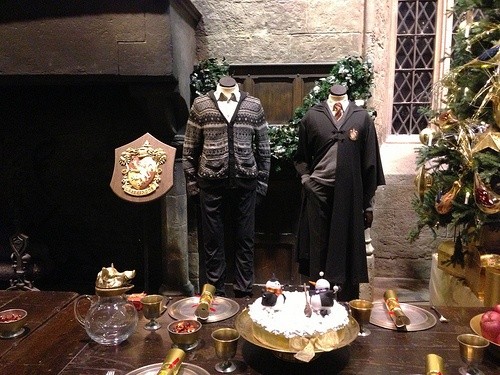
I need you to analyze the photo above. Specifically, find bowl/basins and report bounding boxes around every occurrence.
[470,313,500,346]
[236,306,360,359]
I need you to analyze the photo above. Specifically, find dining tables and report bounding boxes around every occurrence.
[0,295,500,375]
[0,288,77,357]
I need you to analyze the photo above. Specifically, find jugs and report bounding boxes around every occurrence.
[74,262,139,347]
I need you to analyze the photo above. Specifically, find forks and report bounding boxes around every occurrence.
[430,306,450,323]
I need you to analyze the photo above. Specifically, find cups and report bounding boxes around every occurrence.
[167,319,202,350]
[0,309,27,339]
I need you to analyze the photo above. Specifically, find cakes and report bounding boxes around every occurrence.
[248,271,349,350]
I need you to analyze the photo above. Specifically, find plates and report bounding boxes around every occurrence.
[125,362,211,375]
[167,295,239,323]
[369,302,437,332]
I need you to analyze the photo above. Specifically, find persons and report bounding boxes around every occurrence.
[294,85,386,302]
[182,77,270,297]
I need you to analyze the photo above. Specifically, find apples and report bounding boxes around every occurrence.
[480,304,500,344]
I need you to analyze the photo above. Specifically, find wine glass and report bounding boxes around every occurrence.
[211,328,240,373]
[455,334,489,374]
[139,295,163,330]
[349,300,374,335]
